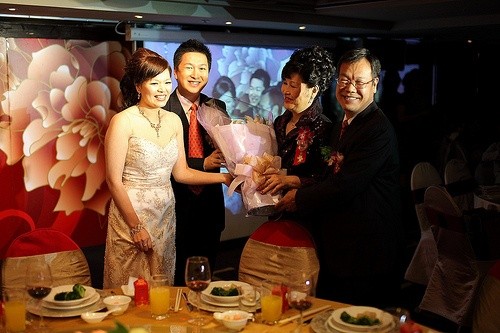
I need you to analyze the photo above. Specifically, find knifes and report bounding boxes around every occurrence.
[181,293,193,312]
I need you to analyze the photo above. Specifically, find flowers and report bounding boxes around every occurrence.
[195,94,288,216]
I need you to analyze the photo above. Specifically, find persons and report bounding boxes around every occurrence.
[103,47,242,292]
[255,44,423,314]
[161,38,226,289]
[211,68,285,128]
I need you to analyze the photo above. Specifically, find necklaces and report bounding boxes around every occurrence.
[135,100,162,140]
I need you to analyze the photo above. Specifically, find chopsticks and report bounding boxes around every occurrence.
[174,289,182,312]
[278,304,331,324]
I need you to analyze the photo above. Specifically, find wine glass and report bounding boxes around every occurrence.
[287,271,314,325]
[25,261,54,333]
[184,256,212,326]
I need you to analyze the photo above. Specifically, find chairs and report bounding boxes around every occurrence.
[1,227,91,304]
[238,220,321,297]
[411,143,500,333]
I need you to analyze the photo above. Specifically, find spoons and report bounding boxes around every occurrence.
[80,308,123,320]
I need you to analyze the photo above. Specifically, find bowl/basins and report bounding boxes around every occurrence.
[121,285,135,296]
[220,311,249,331]
[102,295,132,316]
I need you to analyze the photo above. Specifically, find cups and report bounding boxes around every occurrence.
[261,280,283,324]
[2,289,28,333]
[148,275,170,320]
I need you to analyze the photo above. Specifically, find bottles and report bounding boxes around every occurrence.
[134,273,149,308]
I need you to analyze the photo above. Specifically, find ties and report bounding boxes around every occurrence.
[187,104,204,195]
[342,119,348,134]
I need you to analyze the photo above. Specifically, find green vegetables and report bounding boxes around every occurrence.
[211,287,239,296]
[341,311,380,326]
[54,284,86,300]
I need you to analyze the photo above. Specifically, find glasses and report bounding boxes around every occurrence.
[335,75,374,89]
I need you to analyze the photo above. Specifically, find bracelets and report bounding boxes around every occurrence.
[131,224,142,237]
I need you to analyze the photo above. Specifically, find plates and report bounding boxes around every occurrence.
[310,305,403,333]
[188,280,273,313]
[25,284,105,318]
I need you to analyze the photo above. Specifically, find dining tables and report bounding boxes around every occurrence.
[0,279,438,333]
